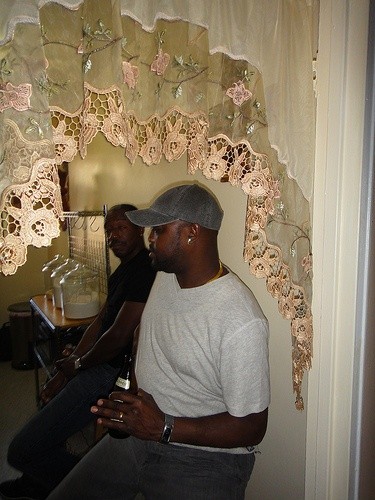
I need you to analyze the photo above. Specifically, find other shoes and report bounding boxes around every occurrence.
[0,475,51,500]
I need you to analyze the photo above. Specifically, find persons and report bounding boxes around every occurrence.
[0,204,156,499]
[43,184,270,500]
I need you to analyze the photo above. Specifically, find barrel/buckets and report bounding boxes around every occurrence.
[6,302,42,370]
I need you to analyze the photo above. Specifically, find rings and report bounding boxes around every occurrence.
[119,412,124,420]
[39,383,49,393]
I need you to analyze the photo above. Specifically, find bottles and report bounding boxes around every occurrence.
[109,353,138,439]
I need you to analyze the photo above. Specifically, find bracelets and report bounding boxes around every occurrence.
[74,357,82,370]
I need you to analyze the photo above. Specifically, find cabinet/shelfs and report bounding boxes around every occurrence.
[28,205,111,409]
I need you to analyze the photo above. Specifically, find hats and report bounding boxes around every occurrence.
[124,183,224,231]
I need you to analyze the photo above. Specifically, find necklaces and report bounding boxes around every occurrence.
[201,259,225,286]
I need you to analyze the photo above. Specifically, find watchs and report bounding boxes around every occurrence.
[160,414,175,445]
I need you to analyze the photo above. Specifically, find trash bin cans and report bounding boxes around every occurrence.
[5,301,40,371]
